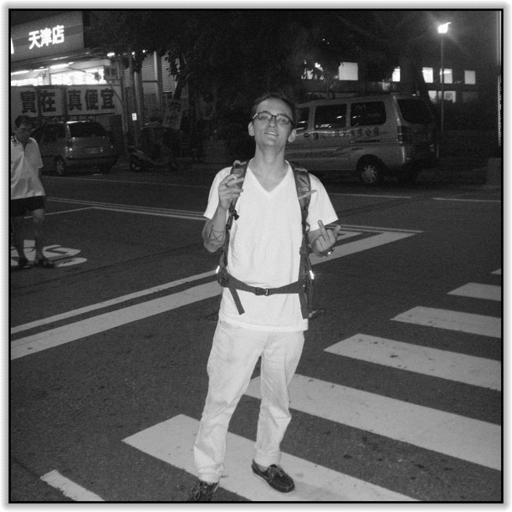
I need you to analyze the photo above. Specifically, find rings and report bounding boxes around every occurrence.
[224,181,231,191]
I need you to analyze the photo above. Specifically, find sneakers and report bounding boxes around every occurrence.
[17,254,54,269]
[187,478,219,502]
[251,459,294,492]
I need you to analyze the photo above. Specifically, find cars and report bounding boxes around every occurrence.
[30,120,118,175]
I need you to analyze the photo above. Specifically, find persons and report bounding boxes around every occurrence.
[185,95,340,501]
[12,114,51,272]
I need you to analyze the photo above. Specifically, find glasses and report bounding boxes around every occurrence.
[252,110,295,129]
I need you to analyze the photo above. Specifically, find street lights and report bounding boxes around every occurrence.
[438,22,451,134]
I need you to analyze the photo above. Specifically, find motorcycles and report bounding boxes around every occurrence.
[126,131,178,175]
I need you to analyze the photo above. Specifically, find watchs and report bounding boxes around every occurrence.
[323,247,334,259]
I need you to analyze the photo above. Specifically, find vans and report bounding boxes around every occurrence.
[284,93,437,187]
